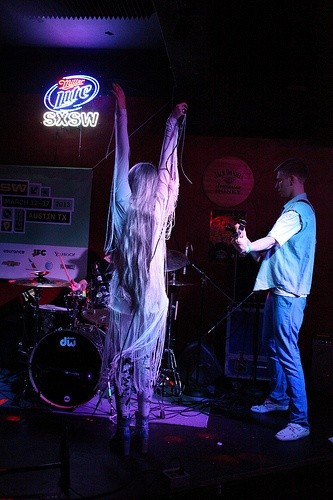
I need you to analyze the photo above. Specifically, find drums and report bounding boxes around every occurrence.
[30,323,105,408]
[17,305,78,355]
[80,280,111,325]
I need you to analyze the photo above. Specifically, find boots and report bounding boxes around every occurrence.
[131,412,150,440]
[110,417,130,452]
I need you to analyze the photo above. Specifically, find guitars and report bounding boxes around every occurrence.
[225,219,269,297]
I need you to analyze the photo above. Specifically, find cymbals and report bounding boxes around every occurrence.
[168,281,195,286]
[104,249,189,272]
[8,271,71,288]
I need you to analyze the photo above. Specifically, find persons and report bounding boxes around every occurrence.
[230,160,316,440]
[48,249,106,308]
[104,81,188,460]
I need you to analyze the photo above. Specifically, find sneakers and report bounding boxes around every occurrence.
[250,399,289,413]
[275,423,310,441]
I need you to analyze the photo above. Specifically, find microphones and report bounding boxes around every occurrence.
[182,244,189,275]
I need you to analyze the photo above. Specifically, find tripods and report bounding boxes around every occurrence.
[163,286,184,406]
